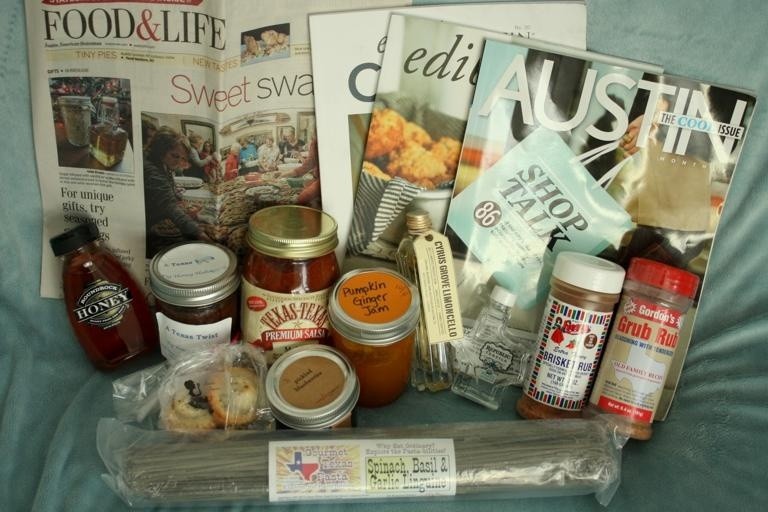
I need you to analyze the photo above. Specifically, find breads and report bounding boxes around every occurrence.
[163,367,259,435]
[363,108,463,189]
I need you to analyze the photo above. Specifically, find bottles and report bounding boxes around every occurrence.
[87,96,127,169]
[450,284,531,411]
[513,249,628,419]
[52,202,471,433]
[582,257,700,442]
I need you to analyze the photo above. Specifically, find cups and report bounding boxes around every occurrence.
[55,93,94,149]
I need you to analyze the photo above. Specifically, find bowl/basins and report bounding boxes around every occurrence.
[282,157,300,165]
[277,163,302,172]
[378,188,453,246]
[287,177,305,188]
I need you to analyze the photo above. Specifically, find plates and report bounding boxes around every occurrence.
[172,176,204,188]
[180,189,213,201]
[243,184,281,198]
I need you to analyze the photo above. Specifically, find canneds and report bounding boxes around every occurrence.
[326,266,421,408]
[239,203,339,370]
[264,344,359,433]
[147,237,240,365]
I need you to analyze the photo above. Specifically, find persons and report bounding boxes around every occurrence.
[144,116,319,247]
